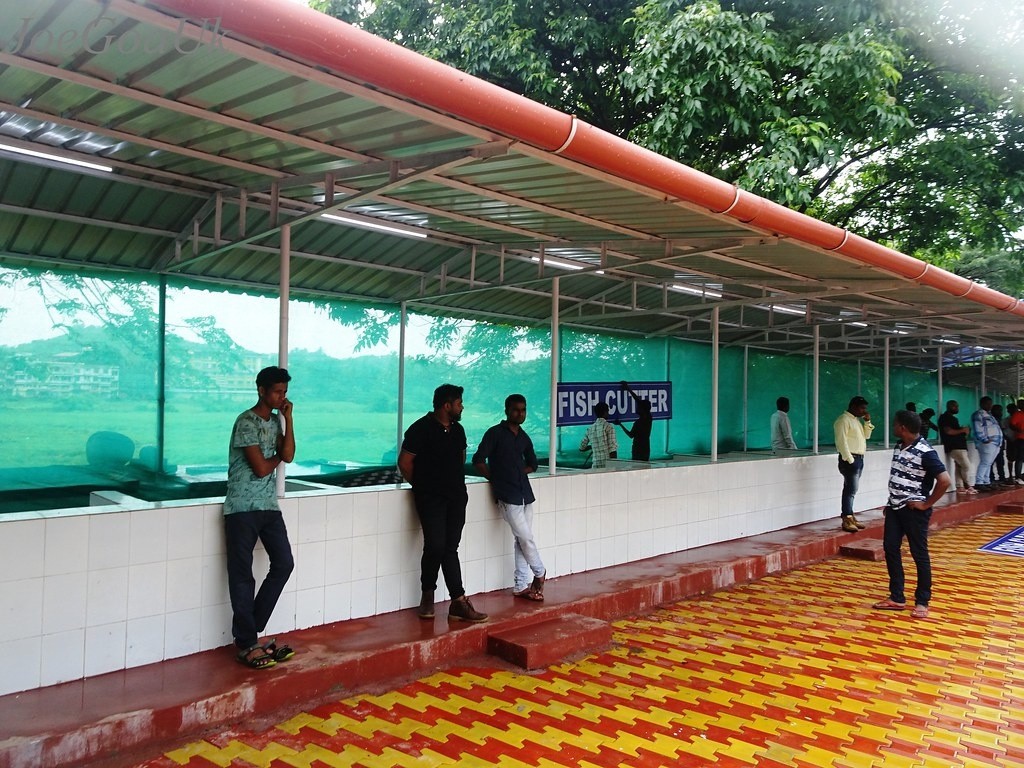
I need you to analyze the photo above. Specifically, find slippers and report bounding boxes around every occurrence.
[515,589,545,601]
[956,488,968,494]
[531,573,545,597]
[966,488,979,495]
[873,599,906,610]
[911,603,927,617]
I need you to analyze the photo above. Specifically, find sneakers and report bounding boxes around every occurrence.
[842,516,858,532]
[418,590,435,617]
[852,516,865,529]
[448,600,489,623]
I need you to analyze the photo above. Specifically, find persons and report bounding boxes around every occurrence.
[937,400,978,494]
[397,384,488,622]
[579,402,618,469]
[223,366,295,668]
[972,396,1024,491]
[613,381,652,460]
[833,396,875,532]
[471,393,546,600]
[906,401,916,413]
[874,410,951,616]
[770,397,798,454]
[918,408,939,440]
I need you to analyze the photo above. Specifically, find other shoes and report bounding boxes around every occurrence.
[975,478,1024,491]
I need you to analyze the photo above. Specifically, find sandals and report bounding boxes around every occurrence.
[236,644,277,669]
[263,642,294,661]
